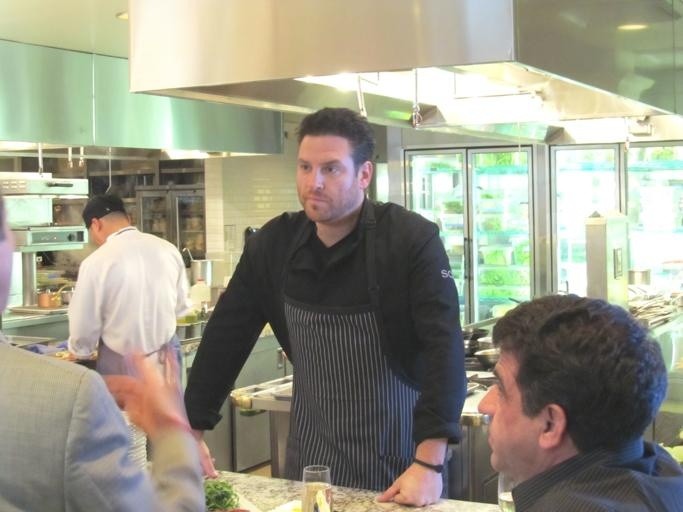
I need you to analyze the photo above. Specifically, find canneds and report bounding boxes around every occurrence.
[48,292,62,308]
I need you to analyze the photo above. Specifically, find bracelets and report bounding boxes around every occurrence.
[411,456,445,473]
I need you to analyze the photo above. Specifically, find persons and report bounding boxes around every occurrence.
[178,104,468,509]
[0,196,205,512]
[55,195,192,400]
[476,293,681,512]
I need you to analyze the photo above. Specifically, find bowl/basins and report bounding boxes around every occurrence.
[461,326,501,367]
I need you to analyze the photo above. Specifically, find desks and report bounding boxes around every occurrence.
[203,470,502,512]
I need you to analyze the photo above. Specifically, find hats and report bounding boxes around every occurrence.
[81,195,124,229]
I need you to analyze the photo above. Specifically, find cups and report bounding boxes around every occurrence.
[497,471,518,511]
[37,284,74,308]
[302,464,333,512]
[190,259,213,290]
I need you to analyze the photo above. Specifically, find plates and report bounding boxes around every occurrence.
[121,412,150,475]
